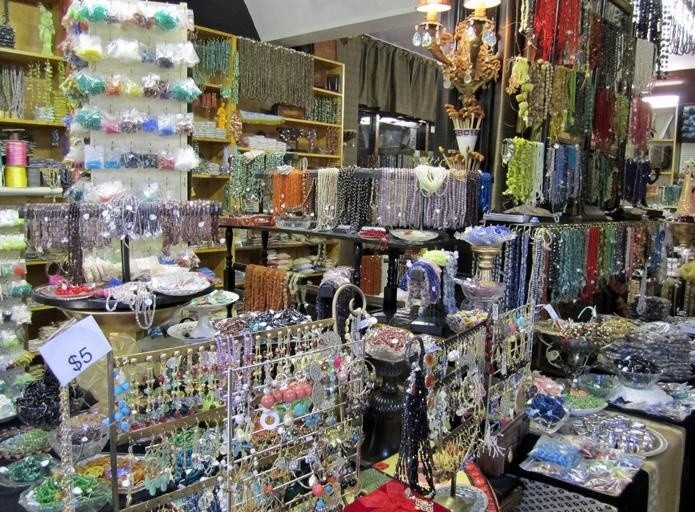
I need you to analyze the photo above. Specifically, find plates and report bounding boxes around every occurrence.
[568,402,610,417]
[390,228,439,245]
[0,454,60,489]
[167,321,222,344]
[634,426,669,457]
[32,283,94,301]
[429,483,489,512]
[147,281,211,296]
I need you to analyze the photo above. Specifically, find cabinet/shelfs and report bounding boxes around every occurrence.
[0,1,81,286]
[185,24,347,288]
[219,149,494,337]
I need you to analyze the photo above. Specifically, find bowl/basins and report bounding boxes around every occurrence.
[612,368,664,389]
[577,373,620,399]
[14,397,84,430]
[47,422,110,461]
[18,474,113,512]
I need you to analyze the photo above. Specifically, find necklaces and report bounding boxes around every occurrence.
[0,1,694,512]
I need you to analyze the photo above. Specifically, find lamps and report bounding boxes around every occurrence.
[413,0,509,171]
